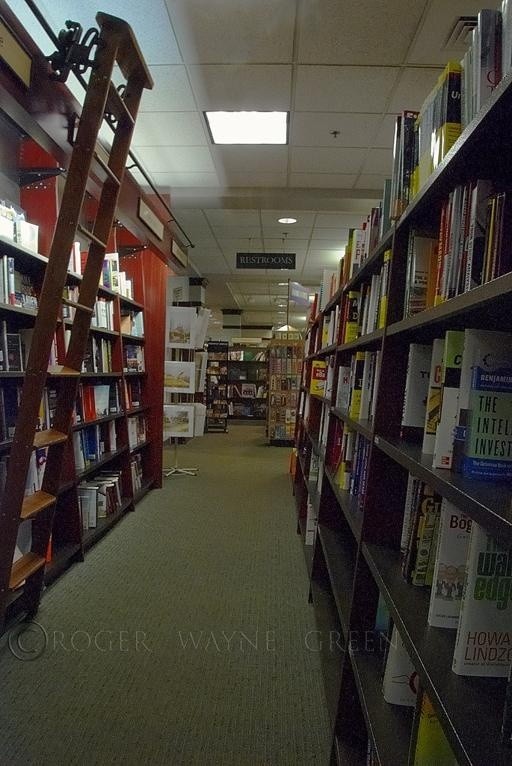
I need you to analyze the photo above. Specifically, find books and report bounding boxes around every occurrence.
[163,305,305,442]
[288,0,512,764]
[0,200,149,595]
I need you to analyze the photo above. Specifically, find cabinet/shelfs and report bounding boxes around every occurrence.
[1,1,229,633]
[266,68,512,765]
[227,346,268,417]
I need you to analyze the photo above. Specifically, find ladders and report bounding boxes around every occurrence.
[0,11,154,636]
